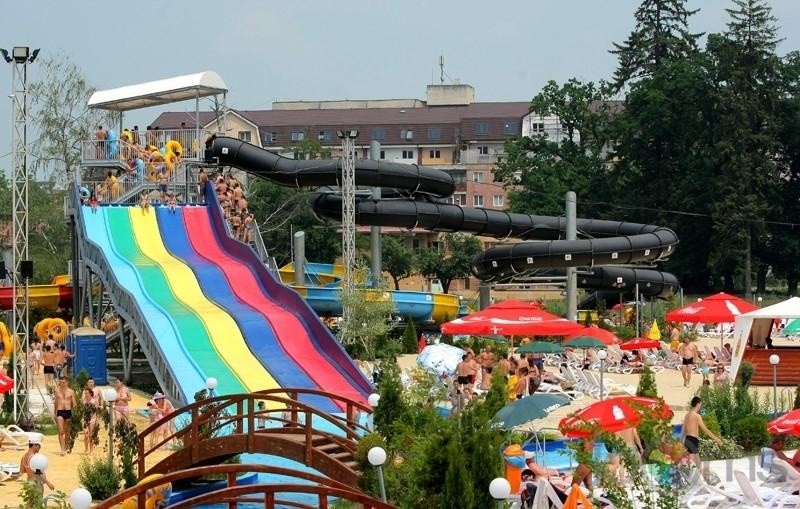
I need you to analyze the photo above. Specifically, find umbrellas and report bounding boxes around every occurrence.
[487,392,573,473]
[667,291,760,363]
[515,324,660,378]
[439,296,585,359]
[768,407,800,438]
[783,319,799,336]
[560,396,672,482]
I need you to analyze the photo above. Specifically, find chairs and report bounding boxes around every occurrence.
[550,365,628,403]
[0,425,46,451]
[0,462,25,484]
[519,446,799,509]
[542,337,731,376]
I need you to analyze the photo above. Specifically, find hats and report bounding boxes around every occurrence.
[151,392,166,400]
[28,439,42,449]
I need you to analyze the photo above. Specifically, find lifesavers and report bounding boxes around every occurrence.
[120,130,182,183]
[34,317,67,343]
[120,474,173,508]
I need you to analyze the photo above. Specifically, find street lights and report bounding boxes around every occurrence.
[769,354,780,421]
[69,487,93,509]
[596,346,606,407]
[0,46,43,431]
[104,383,118,479]
[29,453,49,490]
[489,475,511,509]
[459,295,464,306]
[757,294,762,308]
[364,445,388,505]
[335,127,359,349]
[365,391,379,436]
[205,377,217,398]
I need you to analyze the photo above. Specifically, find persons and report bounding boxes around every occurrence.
[19,333,176,508]
[521,395,724,509]
[558,305,734,392]
[451,338,546,415]
[281,401,304,428]
[257,401,271,428]
[81,120,255,247]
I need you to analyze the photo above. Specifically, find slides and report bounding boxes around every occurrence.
[280,264,477,332]
[81,203,379,419]
[206,133,680,311]
[0,272,97,310]
